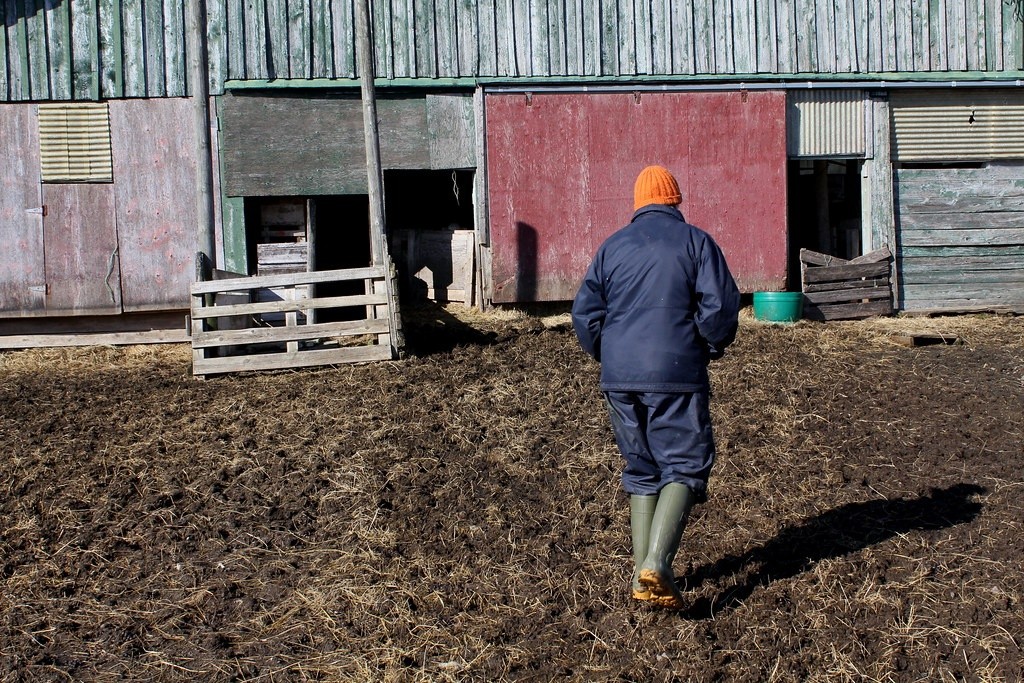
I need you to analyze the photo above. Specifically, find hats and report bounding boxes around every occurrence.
[633,166,682,212]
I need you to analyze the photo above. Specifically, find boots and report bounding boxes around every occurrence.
[638,482,689,607]
[631,493,658,600]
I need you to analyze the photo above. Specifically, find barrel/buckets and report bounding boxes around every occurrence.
[753,291,804,323]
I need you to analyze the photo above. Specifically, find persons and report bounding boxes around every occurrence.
[570,166,741,608]
